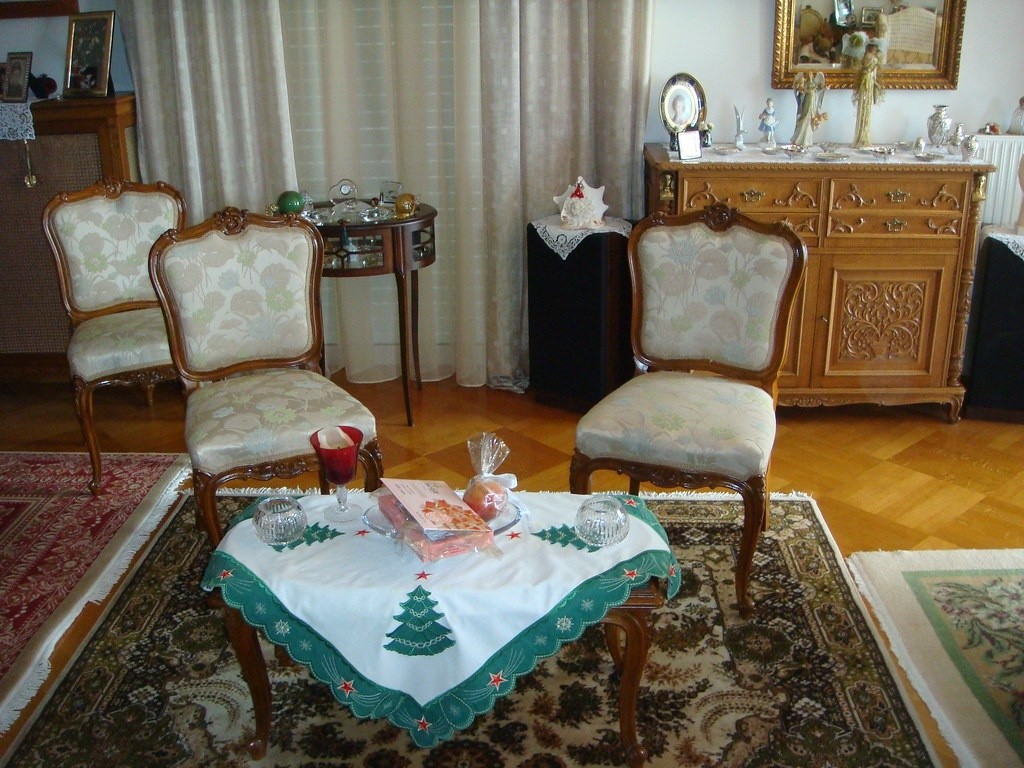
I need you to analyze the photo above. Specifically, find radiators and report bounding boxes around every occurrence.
[966,131,1024,225]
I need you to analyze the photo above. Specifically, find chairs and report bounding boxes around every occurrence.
[42,177,206,493]
[568,201,808,623]
[879,6,943,66]
[149,206,386,549]
[799,8,823,41]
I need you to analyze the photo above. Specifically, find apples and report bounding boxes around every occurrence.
[463,481,506,521]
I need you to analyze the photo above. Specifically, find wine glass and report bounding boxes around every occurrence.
[309,425,364,521]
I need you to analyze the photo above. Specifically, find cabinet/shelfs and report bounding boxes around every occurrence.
[643,143,997,423]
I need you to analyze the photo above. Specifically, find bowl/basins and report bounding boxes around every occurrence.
[818,141,843,152]
[870,147,899,160]
[781,144,810,160]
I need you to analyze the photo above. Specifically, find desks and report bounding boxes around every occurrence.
[526,213,636,416]
[0,90,141,384]
[199,487,681,766]
[273,198,437,429]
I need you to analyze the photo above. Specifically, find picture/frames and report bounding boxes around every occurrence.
[3,52,43,103]
[862,7,884,25]
[657,71,712,152]
[770,0,967,90]
[63,10,116,99]
[0,63,7,100]
[833,0,854,24]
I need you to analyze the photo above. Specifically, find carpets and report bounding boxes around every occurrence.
[0,448,192,737]
[0,480,942,768]
[844,547,1024,768]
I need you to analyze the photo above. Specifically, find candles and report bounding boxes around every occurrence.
[310,425,365,522]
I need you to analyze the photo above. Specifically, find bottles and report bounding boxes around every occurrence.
[912,138,925,154]
[574,496,630,547]
[962,134,979,162]
[927,105,952,148]
[252,496,308,545]
[947,124,965,155]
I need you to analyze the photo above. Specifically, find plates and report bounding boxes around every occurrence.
[714,147,741,154]
[914,153,944,162]
[763,147,783,155]
[362,500,521,539]
[815,152,851,162]
